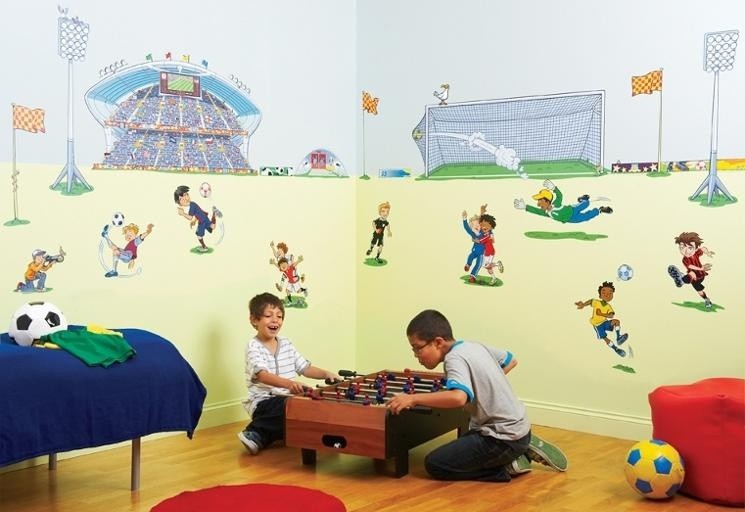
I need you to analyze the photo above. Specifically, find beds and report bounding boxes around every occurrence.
[0,325,208,492]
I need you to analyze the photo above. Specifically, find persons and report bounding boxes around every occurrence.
[575,281,629,357]
[269,241,305,283]
[276,255,308,307]
[15,249,56,292]
[668,232,716,308]
[174,186,223,252]
[366,202,393,264]
[472,214,503,285]
[386,310,569,483]
[101,223,153,277]
[462,203,495,283]
[514,180,613,223]
[237,292,342,457]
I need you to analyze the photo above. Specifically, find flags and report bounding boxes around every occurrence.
[632,71,662,96]
[12,104,45,134]
[146,52,208,67]
[361,91,380,115]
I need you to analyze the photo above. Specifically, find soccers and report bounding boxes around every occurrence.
[113,215,125,226]
[15,302,65,345]
[201,184,211,199]
[618,265,634,281]
[625,439,685,501]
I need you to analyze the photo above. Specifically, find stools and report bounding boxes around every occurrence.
[149,483,346,512]
[648,378,744,507]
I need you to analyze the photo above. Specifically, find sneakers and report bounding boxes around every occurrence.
[504,454,533,476]
[526,433,568,472]
[237,429,263,455]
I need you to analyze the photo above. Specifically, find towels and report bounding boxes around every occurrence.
[40,329,136,369]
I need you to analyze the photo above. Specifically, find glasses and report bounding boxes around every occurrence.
[412,341,431,354]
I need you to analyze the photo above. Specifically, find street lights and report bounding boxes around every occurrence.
[689,30,739,204]
[50,16,92,194]
